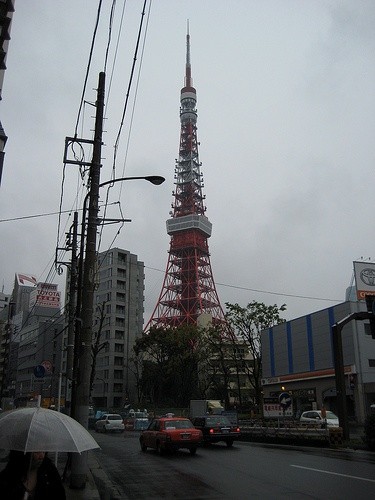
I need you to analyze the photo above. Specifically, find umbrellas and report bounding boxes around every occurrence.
[0,394,101,500]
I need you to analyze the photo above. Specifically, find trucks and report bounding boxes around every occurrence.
[189,400,225,417]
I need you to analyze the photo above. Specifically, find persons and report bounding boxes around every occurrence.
[0,432,66,500]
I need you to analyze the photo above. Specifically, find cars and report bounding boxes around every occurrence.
[139,413,203,457]
[299,410,339,428]
[87,404,150,430]
[95,413,125,433]
[191,416,241,449]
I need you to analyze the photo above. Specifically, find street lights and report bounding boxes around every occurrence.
[63,175,166,483]
